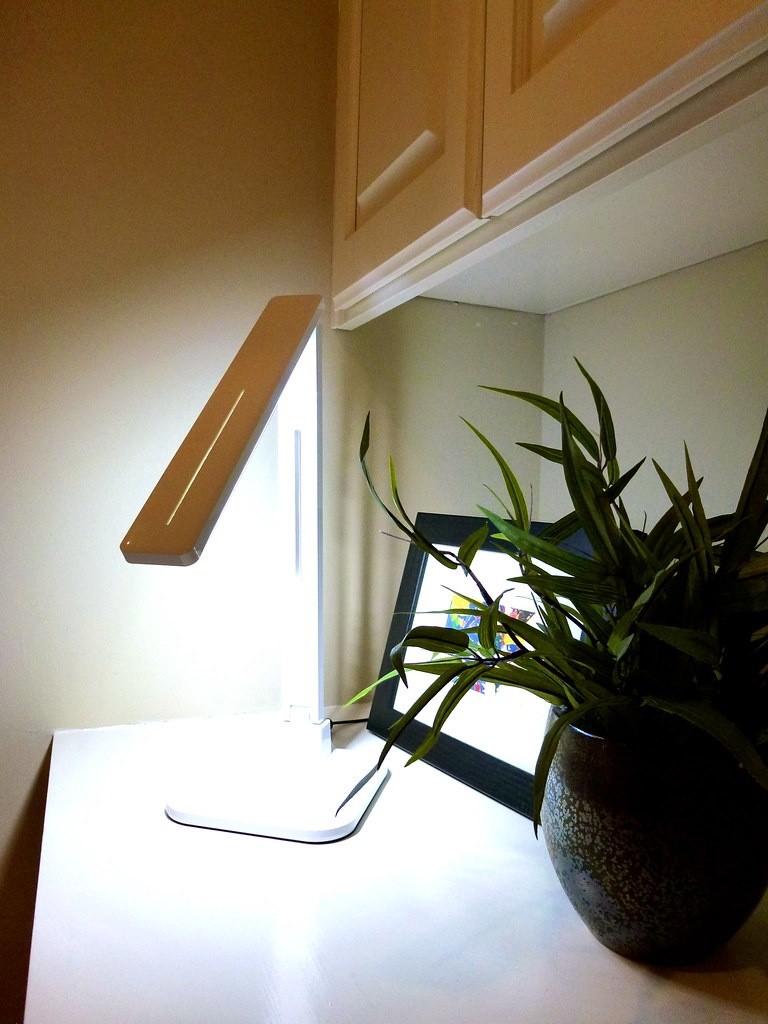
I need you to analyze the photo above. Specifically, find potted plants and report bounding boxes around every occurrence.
[314,353,768,976]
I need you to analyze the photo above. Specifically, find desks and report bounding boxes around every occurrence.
[23,717,768,1024]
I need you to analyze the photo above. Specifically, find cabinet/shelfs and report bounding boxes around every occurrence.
[325,0,768,334]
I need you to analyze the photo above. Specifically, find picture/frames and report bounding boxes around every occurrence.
[364,511,573,826]
